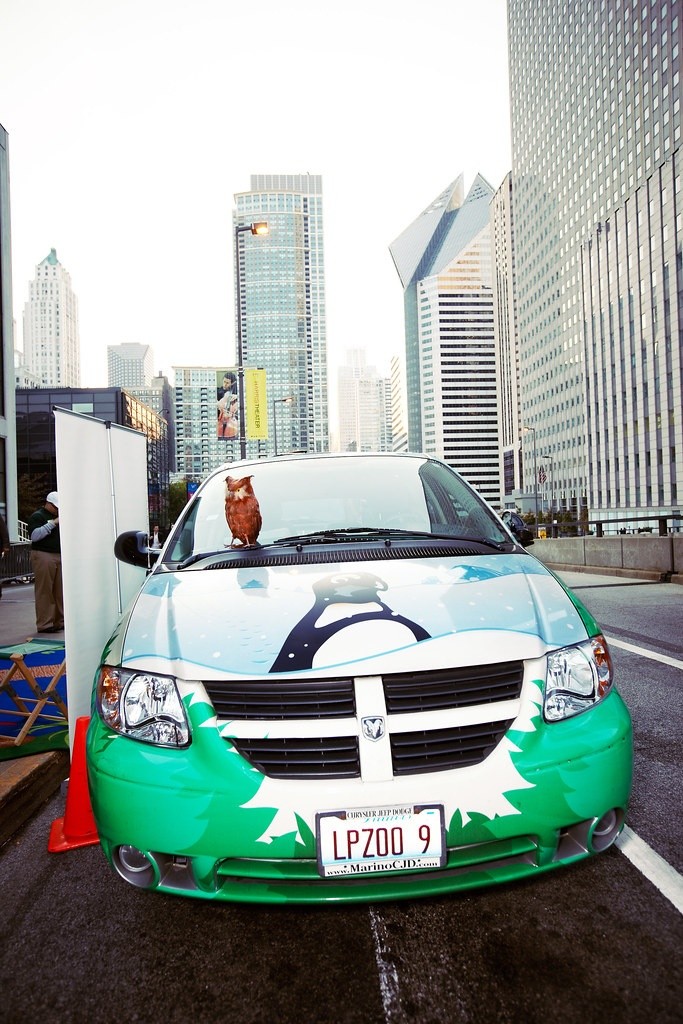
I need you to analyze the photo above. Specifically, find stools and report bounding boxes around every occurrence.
[0,638,68,747]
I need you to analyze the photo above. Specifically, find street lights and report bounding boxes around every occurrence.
[542,456,553,538]
[235,222,268,460]
[524,427,538,538]
[273,398,293,457]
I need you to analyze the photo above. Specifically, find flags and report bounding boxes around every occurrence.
[538,466,547,484]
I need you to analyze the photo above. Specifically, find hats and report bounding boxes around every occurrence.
[47,491,58,508]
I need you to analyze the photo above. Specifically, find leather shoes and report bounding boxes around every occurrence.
[57,627,64,630]
[38,626,62,633]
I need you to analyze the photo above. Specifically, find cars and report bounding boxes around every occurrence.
[85,452,634,903]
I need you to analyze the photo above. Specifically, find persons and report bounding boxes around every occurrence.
[149,523,175,568]
[620,526,652,534]
[0,514,10,598]
[217,373,239,439]
[29,491,65,634]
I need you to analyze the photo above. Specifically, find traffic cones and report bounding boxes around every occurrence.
[48,715,101,852]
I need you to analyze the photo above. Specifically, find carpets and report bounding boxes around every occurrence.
[0,643,70,761]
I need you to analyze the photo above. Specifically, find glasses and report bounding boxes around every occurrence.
[50,502,58,509]
